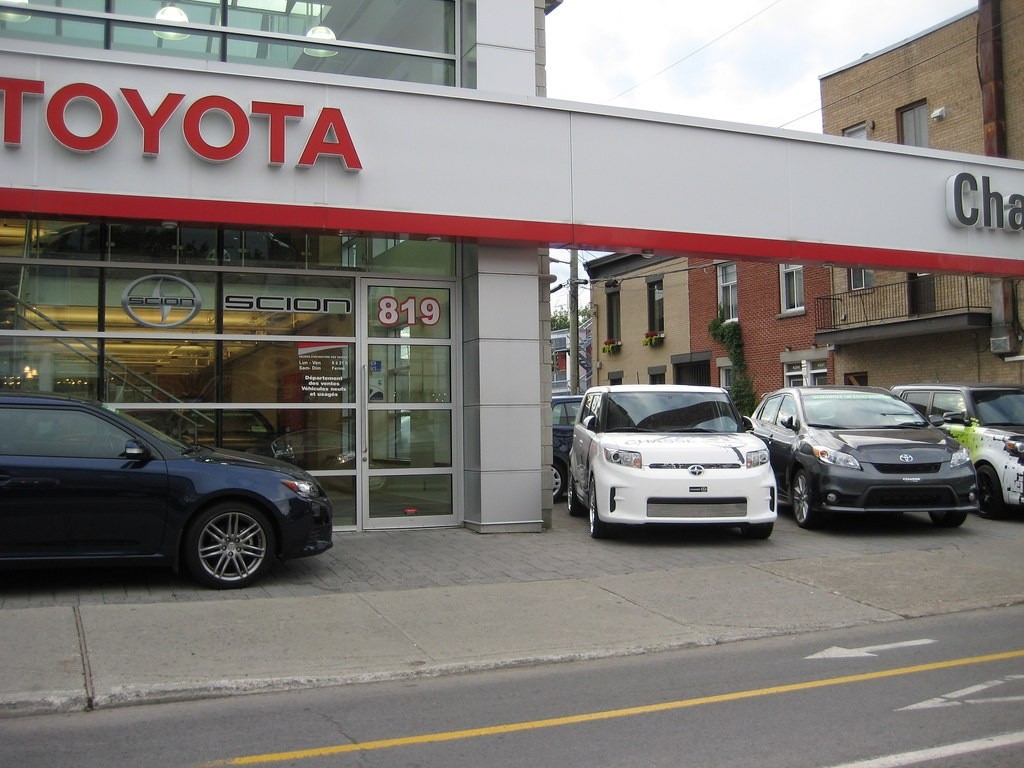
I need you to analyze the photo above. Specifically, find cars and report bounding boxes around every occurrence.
[172,409,294,464]
[551,395,585,503]
[889,384,1024,520]
[1,390,334,591]
[567,384,778,541]
[80,225,295,260]
[746,385,981,531]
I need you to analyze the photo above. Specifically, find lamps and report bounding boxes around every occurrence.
[302,0,339,58]
[152,0,191,42]
[930,108,946,122]
[0,1,32,23]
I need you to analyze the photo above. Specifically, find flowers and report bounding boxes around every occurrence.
[602,340,618,352]
[641,331,661,347]
